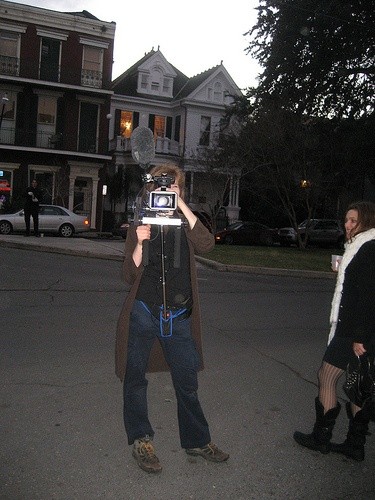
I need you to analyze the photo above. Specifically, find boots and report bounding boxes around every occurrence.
[328,401,371,462]
[293,396,341,455]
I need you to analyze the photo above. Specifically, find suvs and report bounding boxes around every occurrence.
[277,218,345,250]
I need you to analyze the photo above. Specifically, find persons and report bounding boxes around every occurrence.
[22,179,42,237]
[114,165,230,473]
[294,201,375,462]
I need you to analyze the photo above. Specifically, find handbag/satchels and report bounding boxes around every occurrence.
[342,357,375,407]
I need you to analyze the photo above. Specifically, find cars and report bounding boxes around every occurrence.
[0,204,90,235]
[112,210,213,240]
[214,221,278,247]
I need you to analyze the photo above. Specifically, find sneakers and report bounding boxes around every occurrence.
[186,442,230,462]
[132,435,162,473]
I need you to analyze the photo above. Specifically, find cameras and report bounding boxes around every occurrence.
[144,173,178,211]
